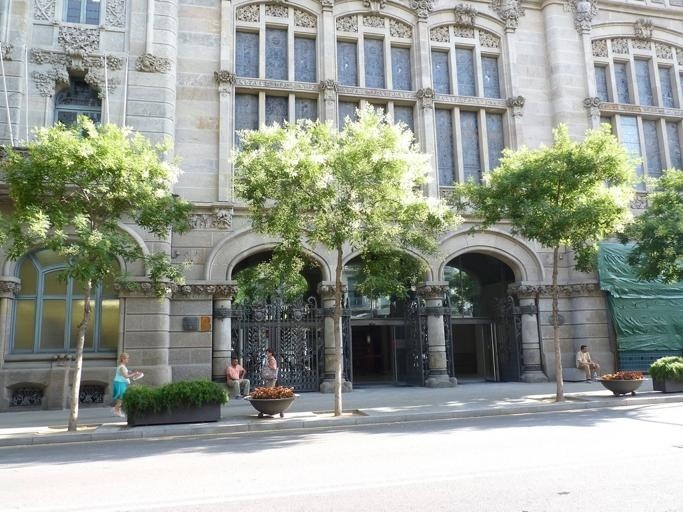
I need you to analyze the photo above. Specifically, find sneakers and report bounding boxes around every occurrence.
[234,395,251,400]
[587,379,594,384]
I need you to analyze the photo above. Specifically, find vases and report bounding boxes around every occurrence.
[245,397,299,417]
[598,379,648,393]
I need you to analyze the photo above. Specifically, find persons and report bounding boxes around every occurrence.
[265,348,279,387]
[226,356,250,399]
[113,352,140,417]
[576,345,601,383]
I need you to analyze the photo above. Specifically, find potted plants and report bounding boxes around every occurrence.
[121,380,226,426]
[649,356,683,392]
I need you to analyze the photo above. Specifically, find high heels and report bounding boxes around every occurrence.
[112,407,126,418]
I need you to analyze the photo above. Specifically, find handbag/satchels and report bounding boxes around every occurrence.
[262,367,278,379]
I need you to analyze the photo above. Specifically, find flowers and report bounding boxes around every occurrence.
[250,385,297,398]
[601,370,644,380]
